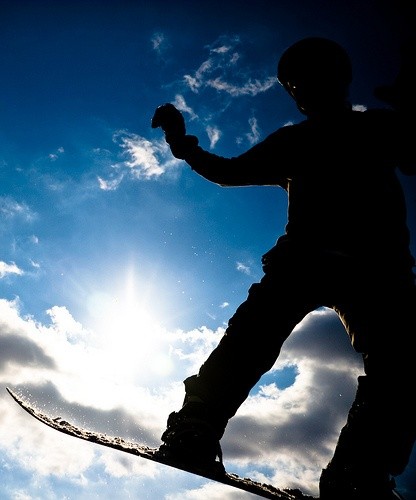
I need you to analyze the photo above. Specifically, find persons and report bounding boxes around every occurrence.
[149,33,416,500]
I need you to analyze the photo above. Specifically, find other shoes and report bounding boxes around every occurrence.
[164,408,228,471]
[320,465,407,500]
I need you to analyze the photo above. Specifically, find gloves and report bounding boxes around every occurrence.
[150,103,186,145]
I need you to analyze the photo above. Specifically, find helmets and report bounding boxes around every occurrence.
[278,37,353,87]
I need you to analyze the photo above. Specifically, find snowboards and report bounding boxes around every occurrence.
[5,386,416,500]
